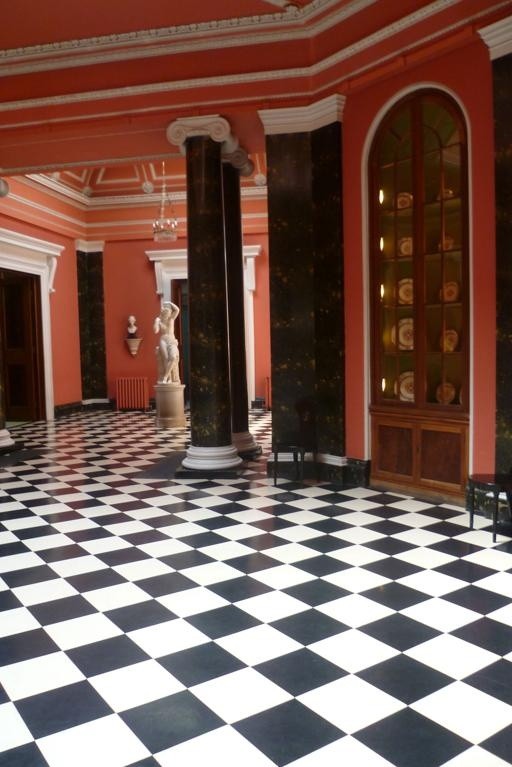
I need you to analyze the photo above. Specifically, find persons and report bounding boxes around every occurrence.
[127,316,137,333]
[153,301,181,384]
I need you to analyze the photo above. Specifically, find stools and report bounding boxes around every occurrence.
[467,473,512,542]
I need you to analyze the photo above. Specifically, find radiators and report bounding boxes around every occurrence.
[116,376,149,412]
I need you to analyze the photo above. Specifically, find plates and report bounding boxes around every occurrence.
[388,187,465,405]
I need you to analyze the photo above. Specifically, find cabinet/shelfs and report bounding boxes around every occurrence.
[360,81,473,499]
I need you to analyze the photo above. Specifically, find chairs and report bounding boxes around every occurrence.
[274,400,318,487]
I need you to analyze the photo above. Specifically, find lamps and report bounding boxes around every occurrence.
[152,160,178,243]
